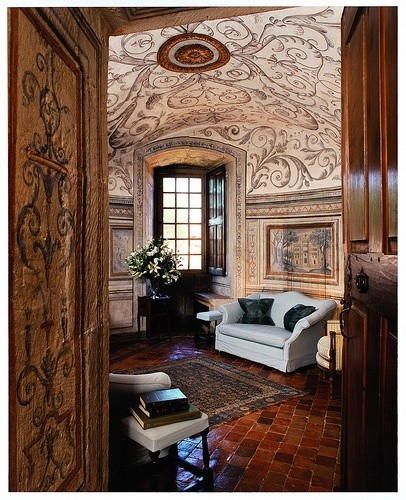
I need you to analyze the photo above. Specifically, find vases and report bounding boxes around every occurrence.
[151,285,170,298]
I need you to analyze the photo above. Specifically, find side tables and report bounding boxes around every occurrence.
[123,411,218,492]
[196,311,220,349]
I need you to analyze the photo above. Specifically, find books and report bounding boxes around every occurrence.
[128,387,202,431]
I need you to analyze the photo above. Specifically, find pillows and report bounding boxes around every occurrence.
[284,303,315,332]
[237,297,275,326]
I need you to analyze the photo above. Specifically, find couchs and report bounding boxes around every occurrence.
[109,372,172,469]
[215,290,336,374]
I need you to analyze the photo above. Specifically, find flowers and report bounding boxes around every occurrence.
[123,237,184,299]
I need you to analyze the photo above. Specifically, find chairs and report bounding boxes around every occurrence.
[316,319,344,400]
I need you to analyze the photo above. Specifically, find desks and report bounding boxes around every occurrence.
[194,292,229,334]
[137,297,175,346]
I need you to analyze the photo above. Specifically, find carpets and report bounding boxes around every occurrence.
[115,356,308,439]
